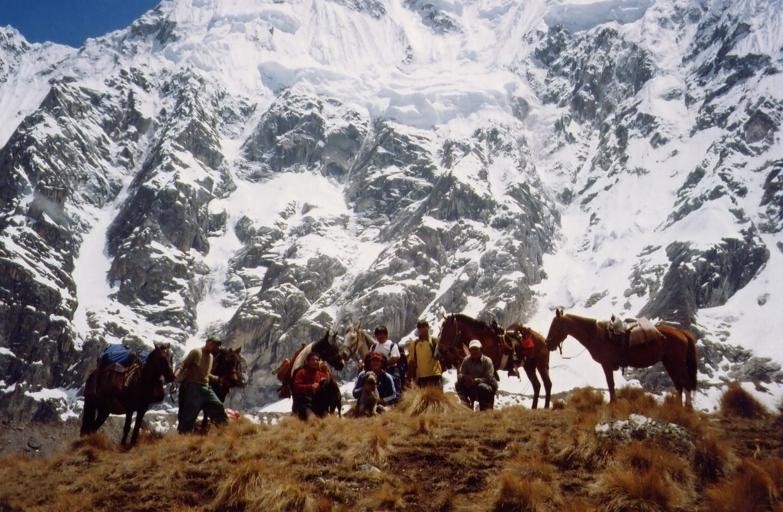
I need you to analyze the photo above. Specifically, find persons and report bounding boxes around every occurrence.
[454,339,499,412]
[173,335,230,435]
[370,325,401,393]
[292,351,331,427]
[352,351,401,405]
[407,318,444,394]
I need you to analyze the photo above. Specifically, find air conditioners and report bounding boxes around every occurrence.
[354,370,387,419]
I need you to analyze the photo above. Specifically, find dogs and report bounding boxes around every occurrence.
[342,321,410,384]
[193,346,245,434]
[545,308,697,408]
[276,329,344,418]
[433,311,552,411]
[79,341,176,449]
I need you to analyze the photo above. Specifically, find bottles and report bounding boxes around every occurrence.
[369,350,383,361]
[206,335,224,346]
[468,338,483,351]
[375,326,389,338]
